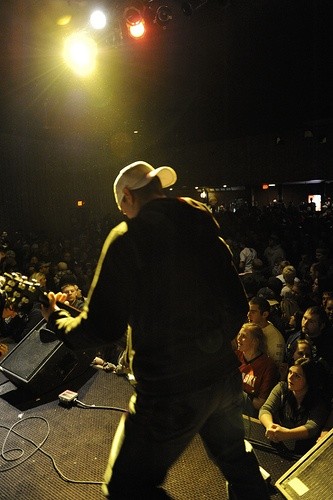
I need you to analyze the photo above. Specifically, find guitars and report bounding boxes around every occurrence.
[0,273,83,316]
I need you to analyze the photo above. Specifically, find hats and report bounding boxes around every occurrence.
[257,286,279,307]
[112,160,176,212]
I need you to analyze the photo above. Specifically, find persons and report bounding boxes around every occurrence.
[38,161,272,500]
[258,357,328,443]
[0,178,332,390]
[231,322,277,419]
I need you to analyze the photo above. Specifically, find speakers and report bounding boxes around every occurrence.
[275,428,333,500]
[0,317,86,395]
